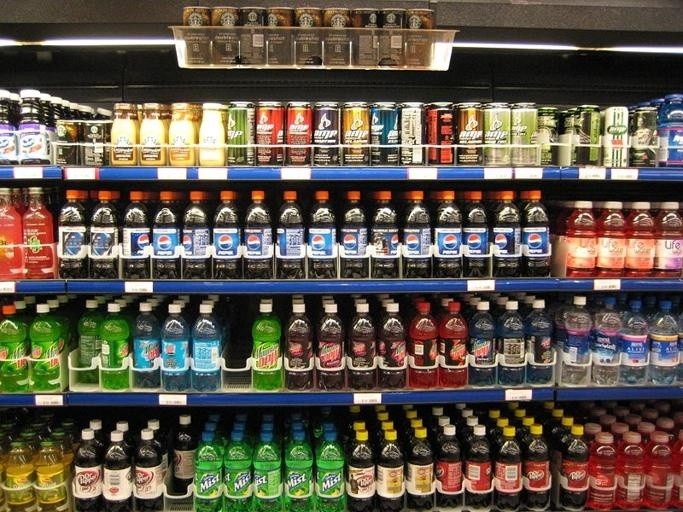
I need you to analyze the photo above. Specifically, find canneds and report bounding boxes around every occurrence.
[372,102,400,168]
[602,105,630,168]
[398,102,426,165]
[225,101,256,165]
[313,101,340,166]
[483,102,511,166]
[426,101,455,164]
[558,108,580,168]
[650,98,665,107]
[577,104,602,167]
[511,102,538,168]
[285,101,314,165]
[54,120,107,166]
[638,100,650,107]
[255,101,285,167]
[456,101,484,165]
[538,107,559,166]
[341,101,371,166]
[184,7,433,66]
[659,94,683,167]
[631,106,656,166]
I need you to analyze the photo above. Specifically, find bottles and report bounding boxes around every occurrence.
[255,296,683,393]
[1,295,220,389]
[0,404,682,511]
[0,87,229,168]
[628,94,681,169]
[0,185,683,285]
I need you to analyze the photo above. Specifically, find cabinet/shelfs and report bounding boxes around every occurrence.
[0,162,68,415]
[554,161,682,405]
[50,153,565,420]
